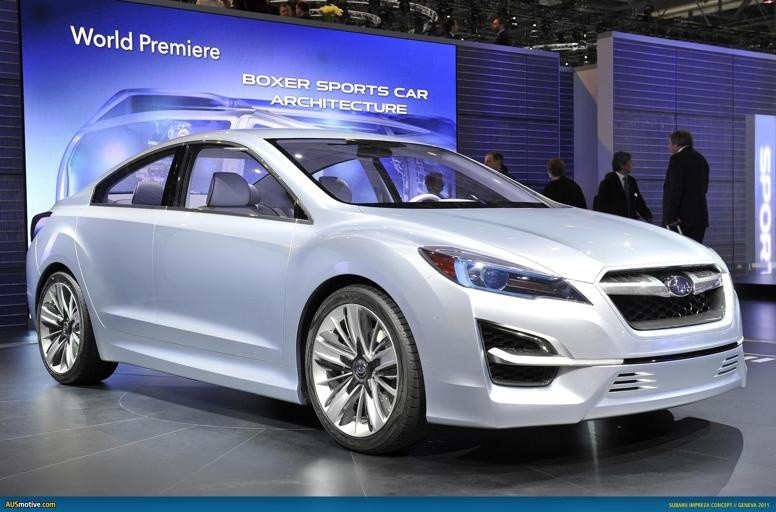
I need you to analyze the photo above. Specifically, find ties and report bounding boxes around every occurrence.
[622,176,632,196]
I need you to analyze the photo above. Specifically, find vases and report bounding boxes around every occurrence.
[329,13,335,24]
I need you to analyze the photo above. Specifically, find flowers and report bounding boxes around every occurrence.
[320,4,343,17]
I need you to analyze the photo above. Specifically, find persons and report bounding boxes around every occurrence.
[441,18,459,39]
[196,0,311,19]
[492,18,511,46]
[543,158,588,210]
[662,131,710,243]
[597,152,654,224]
[425,172,446,199]
[484,151,517,181]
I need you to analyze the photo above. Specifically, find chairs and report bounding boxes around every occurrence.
[196,173,294,218]
[133,180,164,205]
[319,176,352,203]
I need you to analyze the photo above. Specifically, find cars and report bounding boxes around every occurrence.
[23,123,751,457]
[51,86,456,203]
